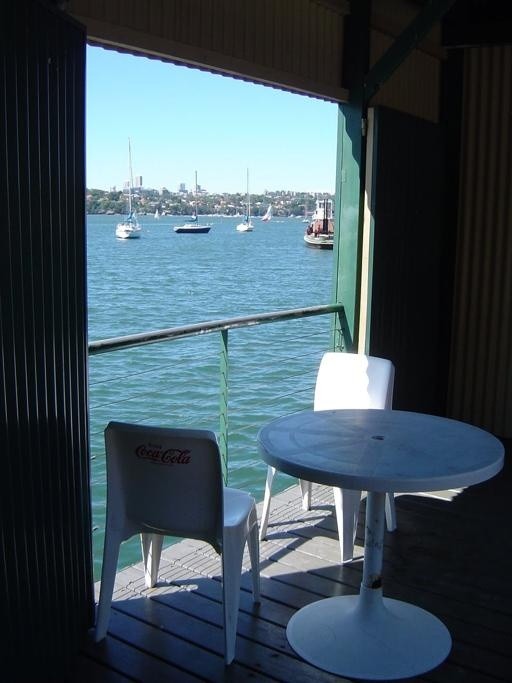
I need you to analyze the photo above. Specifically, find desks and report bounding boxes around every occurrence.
[259,408,505,679]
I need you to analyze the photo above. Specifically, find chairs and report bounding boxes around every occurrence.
[259,352,396,562]
[94,420,262,666]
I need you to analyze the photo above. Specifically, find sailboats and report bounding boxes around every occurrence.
[261,205,273,224]
[114,141,142,238]
[174,171,211,234]
[236,167,254,232]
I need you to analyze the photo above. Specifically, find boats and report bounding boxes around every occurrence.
[303,199,333,249]
[151,210,161,223]
[302,216,310,223]
[184,212,197,222]
